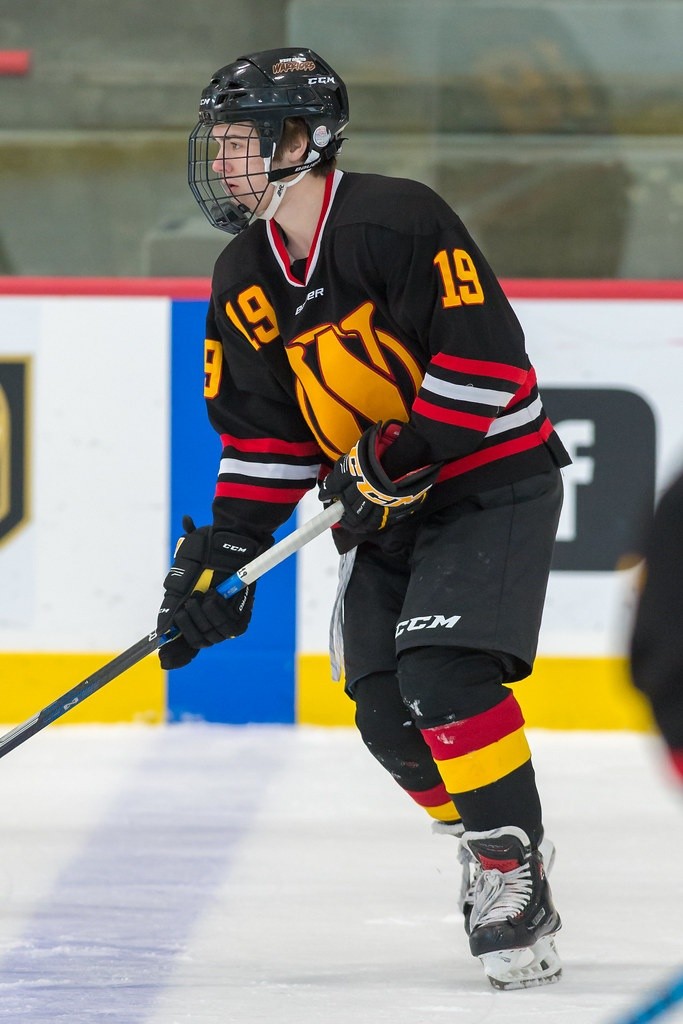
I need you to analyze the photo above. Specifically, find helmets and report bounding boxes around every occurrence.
[186,48,349,234]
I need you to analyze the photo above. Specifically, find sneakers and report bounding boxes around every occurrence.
[462,826,562,991]
[434,821,485,937]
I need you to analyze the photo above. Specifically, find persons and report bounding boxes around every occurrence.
[159,48,574,989]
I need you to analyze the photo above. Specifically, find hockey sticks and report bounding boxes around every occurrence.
[0,498,347,756]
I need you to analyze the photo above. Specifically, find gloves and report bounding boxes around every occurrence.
[318,418,444,535]
[157,515,262,670]
